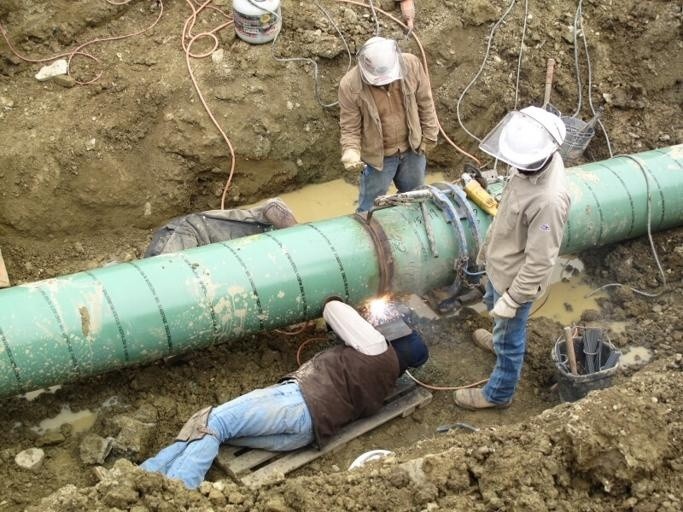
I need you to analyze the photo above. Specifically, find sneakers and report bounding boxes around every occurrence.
[470,326,498,358]
[451,387,512,409]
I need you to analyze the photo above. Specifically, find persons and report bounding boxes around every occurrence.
[138,299,428,492]
[337,35,440,213]
[451,102,569,411]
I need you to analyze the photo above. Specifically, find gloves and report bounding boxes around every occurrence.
[337,149,372,175]
[486,288,523,324]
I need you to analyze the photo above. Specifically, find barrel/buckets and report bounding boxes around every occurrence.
[560,114,595,168]
[552,326,621,403]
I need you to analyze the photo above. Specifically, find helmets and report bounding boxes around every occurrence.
[355,35,406,85]
[475,102,571,171]
